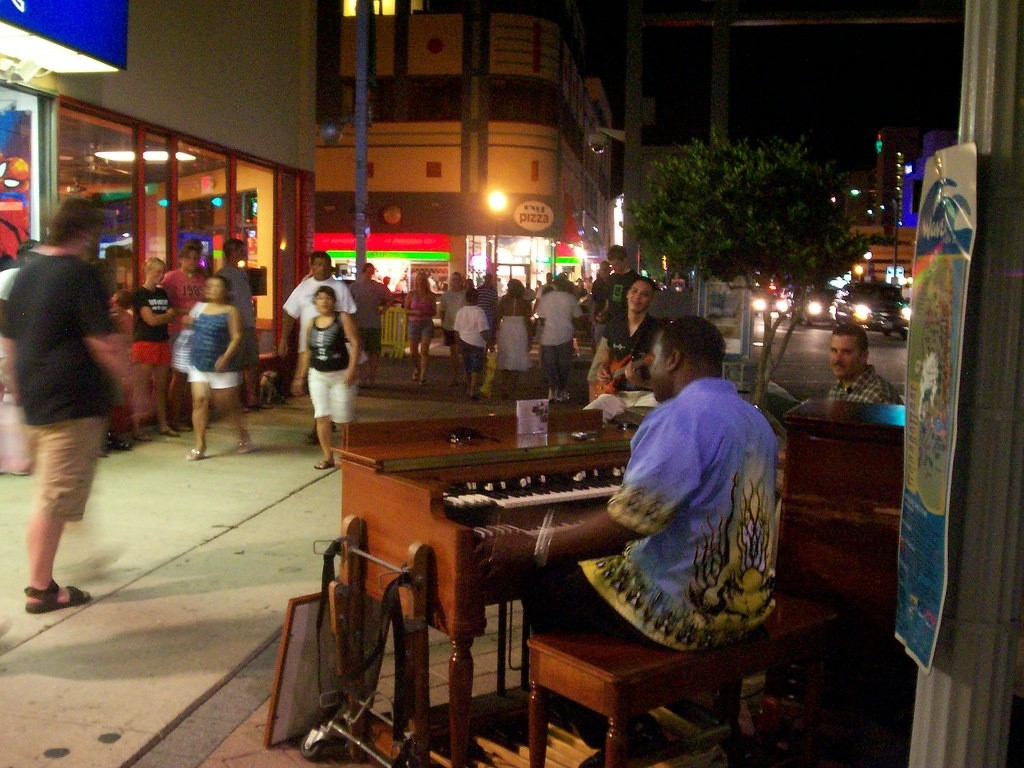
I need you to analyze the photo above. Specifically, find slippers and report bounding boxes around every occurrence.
[314,461,334,469]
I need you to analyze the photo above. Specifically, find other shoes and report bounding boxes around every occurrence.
[412,369,420,380]
[576,750,606,768]
[156,427,179,437]
[131,432,151,441]
[168,423,191,432]
[419,380,425,386]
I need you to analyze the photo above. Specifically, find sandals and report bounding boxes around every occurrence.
[24,580,90,613]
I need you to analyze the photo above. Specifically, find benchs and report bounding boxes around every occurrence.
[526,581,839,768]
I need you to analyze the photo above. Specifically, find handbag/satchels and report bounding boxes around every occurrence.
[170,302,208,373]
[589,353,632,405]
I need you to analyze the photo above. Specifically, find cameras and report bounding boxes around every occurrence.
[571,430,600,440]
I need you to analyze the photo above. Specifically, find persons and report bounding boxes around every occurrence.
[396,274,437,293]
[279,250,358,445]
[405,272,436,387]
[1,194,158,614]
[478,316,778,768]
[218,239,274,413]
[134,258,180,441]
[786,324,907,403]
[349,263,395,390]
[438,245,663,406]
[162,242,216,432]
[290,286,362,471]
[454,288,494,401]
[183,275,252,461]
[588,277,661,402]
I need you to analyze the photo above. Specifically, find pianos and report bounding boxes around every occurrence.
[328,408,638,768]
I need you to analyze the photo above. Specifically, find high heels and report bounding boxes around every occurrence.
[186,449,206,461]
[238,440,252,453]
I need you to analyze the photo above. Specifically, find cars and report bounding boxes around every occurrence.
[753,280,910,340]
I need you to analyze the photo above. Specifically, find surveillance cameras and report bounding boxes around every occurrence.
[589,134,608,154]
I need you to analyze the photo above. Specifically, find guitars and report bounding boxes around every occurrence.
[593,351,654,401]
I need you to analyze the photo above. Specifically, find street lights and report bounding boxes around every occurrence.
[850,189,897,277]
[487,192,508,284]
